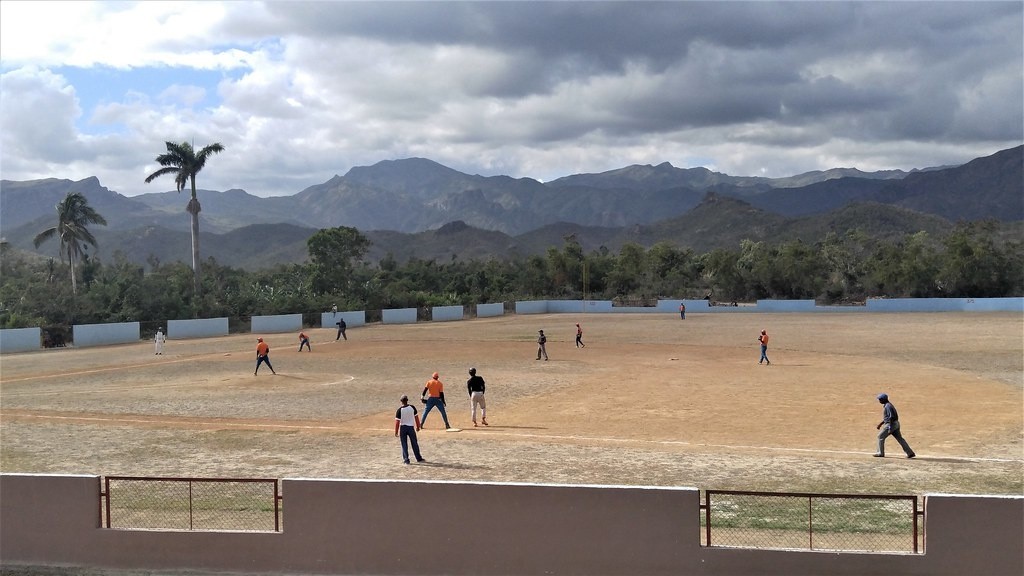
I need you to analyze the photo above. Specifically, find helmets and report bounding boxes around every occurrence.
[761,329,766,335]
[469,367,476,375]
[299,332,303,337]
[257,337,263,343]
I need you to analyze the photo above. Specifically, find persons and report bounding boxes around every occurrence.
[679,302,686,320]
[466,367,489,427]
[536,330,548,361]
[154,326,165,355]
[253,337,276,376]
[420,372,451,429]
[336,318,347,340]
[297,332,311,352]
[872,393,916,459]
[575,322,585,349]
[332,303,337,317]
[394,395,425,464]
[758,329,771,366]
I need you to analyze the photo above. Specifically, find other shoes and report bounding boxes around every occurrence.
[420,423,423,428]
[473,423,479,426]
[544,357,548,361]
[482,421,489,425]
[766,360,769,364]
[417,459,425,462]
[873,453,884,457]
[536,358,540,360]
[446,424,450,428]
[404,461,410,464]
[906,453,915,458]
[582,345,585,348]
[758,361,762,364]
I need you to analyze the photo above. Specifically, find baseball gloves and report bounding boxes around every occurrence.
[422,399,427,403]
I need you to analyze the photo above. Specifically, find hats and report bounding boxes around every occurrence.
[538,330,543,332]
[432,372,440,378]
[877,394,888,400]
[400,395,408,401]
